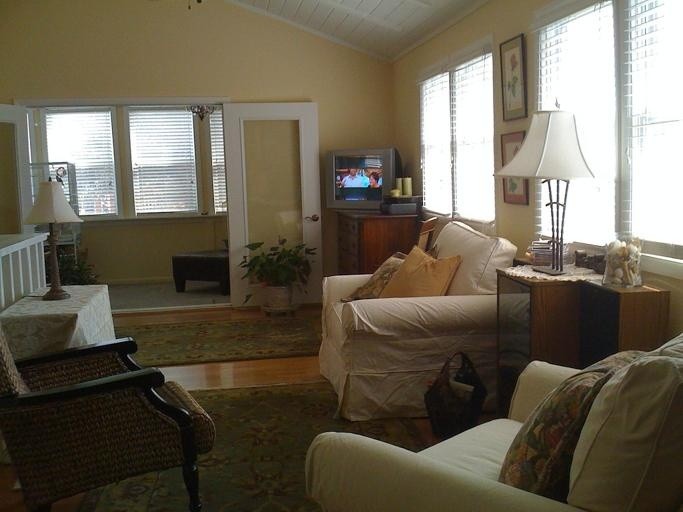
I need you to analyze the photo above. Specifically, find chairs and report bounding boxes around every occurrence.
[318,220,518,423]
[412,215,438,256]
[302,334,682,511]
[0,328,215,510]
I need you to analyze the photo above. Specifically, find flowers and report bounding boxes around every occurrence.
[504,51,518,109]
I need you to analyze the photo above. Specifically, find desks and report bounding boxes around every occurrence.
[2,283,118,359]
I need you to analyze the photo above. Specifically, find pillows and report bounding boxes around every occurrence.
[376,243,463,299]
[338,251,409,303]
[497,347,651,502]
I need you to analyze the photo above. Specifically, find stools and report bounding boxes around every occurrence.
[171,249,230,296]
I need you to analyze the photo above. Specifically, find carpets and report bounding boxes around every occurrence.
[75,377,427,510]
[112,314,322,370]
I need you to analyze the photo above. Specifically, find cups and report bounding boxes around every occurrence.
[390,177,413,197]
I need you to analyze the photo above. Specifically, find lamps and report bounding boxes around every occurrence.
[21,175,86,301]
[184,105,222,122]
[491,110,596,276]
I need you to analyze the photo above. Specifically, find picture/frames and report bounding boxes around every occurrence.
[499,130,528,207]
[499,32,529,123]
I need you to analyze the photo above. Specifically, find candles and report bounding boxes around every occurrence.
[394,177,402,196]
[402,176,412,196]
[389,190,400,196]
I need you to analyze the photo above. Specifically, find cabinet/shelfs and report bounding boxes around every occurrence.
[580,278,671,375]
[335,209,421,275]
[26,161,83,278]
[491,257,593,417]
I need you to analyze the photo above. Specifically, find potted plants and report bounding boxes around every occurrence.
[237,237,319,310]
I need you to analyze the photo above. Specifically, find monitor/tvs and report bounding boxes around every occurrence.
[326,148,401,213]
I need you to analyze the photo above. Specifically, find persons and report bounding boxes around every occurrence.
[340,168,362,199]
[366,172,382,200]
